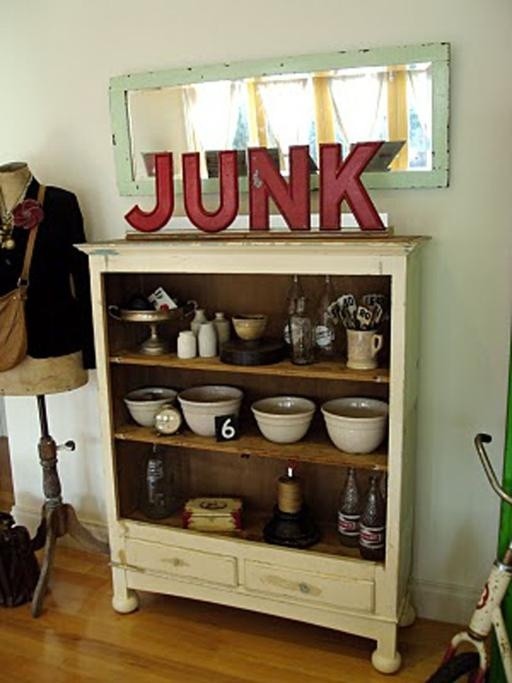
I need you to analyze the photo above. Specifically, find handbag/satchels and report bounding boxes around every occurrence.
[0,286,28,371]
[1,512,42,606]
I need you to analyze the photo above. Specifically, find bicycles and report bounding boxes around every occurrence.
[425,432,512,683]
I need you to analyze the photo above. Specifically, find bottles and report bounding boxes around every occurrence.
[279,274,336,366]
[336,464,386,559]
[176,305,231,362]
[137,441,177,519]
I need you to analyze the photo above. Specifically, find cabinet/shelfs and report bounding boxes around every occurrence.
[71,232,441,672]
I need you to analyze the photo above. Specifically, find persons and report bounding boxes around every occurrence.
[0,160,94,394]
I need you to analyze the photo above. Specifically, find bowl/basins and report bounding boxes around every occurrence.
[228,312,270,341]
[122,383,388,456]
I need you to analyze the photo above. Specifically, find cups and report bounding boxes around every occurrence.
[345,326,384,371]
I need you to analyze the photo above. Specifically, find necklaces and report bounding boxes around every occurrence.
[0,174,34,249]
